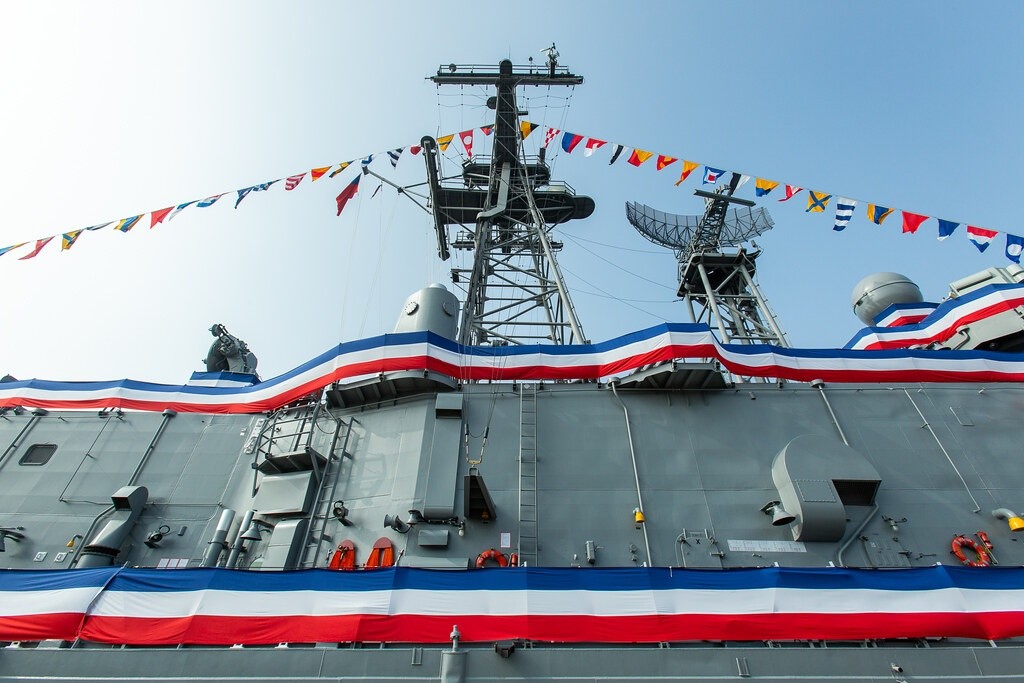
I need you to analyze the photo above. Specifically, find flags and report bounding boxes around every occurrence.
[866,202,1024,263]
[284,142,422,216]
[436,123,495,156]
[730,171,857,231]
[0,213,144,260]
[627,147,726,186]
[520,121,628,166]
[150,179,276,227]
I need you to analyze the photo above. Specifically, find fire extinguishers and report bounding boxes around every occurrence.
[978,530,994,550]
[511,553,518,567]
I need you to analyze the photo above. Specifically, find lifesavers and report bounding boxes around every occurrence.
[952,535,989,567]
[474,549,509,569]
